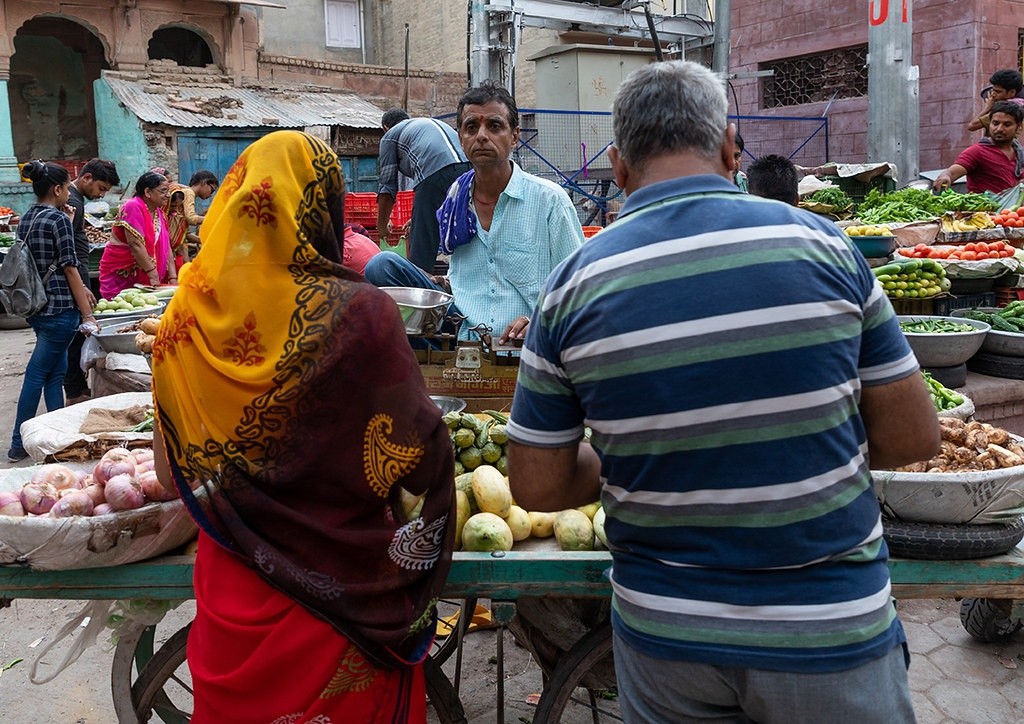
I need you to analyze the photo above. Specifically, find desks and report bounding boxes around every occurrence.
[0,534,1024,724]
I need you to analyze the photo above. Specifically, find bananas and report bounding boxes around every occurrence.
[939,211,996,232]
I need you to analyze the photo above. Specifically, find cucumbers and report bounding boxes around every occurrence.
[871,257,950,298]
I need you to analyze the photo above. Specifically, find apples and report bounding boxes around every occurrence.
[90,291,160,312]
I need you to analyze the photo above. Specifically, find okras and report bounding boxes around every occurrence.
[898,317,981,333]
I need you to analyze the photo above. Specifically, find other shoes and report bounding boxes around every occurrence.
[7,448,29,463]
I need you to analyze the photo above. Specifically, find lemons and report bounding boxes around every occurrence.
[843,226,893,236]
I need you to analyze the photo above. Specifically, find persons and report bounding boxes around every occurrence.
[99,167,220,301]
[64,159,120,407]
[343,79,585,367]
[932,101,1024,194]
[968,70,1024,139]
[505,60,942,723]
[8,158,102,461]
[150,130,457,724]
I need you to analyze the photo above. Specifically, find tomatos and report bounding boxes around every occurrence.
[990,206,1024,228]
[899,241,1017,259]
[0,206,14,216]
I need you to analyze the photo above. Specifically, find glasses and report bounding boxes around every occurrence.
[208,183,215,194]
[155,188,170,193]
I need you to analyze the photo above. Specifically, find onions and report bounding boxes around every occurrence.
[0,447,183,518]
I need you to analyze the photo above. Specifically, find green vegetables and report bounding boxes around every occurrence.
[803,187,853,211]
[859,185,945,215]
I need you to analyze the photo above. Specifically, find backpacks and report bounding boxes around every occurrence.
[0,209,58,318]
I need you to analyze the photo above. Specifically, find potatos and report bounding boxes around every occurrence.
[134,314,163,353]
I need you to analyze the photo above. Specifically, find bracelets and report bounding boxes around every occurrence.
[81,313,93,318]
[167,277,177,281]
[144,266,156,273]
[83,283,86,287]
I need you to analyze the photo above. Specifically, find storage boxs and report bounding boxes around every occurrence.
[342,189,412,254]
[891,289,1024,317]
[580,225,601,240]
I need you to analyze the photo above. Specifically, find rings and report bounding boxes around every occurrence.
[525,316,531,324]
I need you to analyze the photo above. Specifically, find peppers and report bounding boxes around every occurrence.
[927,185,998,213]
[921,370,964,410]
[855,201,938,224]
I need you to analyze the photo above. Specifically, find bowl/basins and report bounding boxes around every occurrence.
[950,307,1024,358]
[94,315,149,327]
[850,235,897,258]
[896,315,992,368]
[377,285,455,335]
[92,301,166,319]
[430,395,467,418]
[91,321,141,356]
[158,296,171,304]
